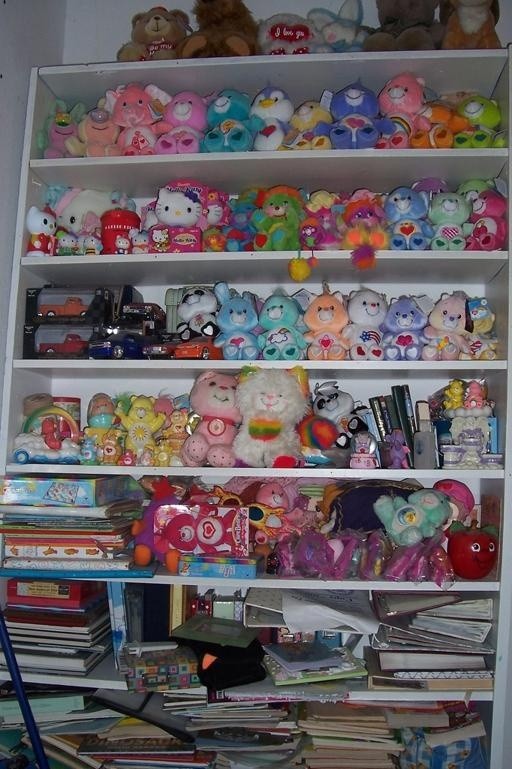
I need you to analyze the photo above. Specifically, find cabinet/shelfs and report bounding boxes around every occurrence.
[0,46,510,769]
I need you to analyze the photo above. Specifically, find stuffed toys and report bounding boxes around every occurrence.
[14,0,506,580]
